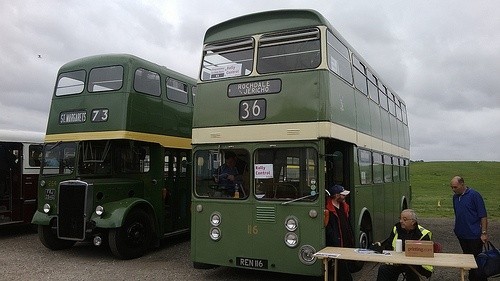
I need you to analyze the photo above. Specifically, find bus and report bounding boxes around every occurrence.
[182,8,412,281]
[1,130,76,230]
[31,55,223,258]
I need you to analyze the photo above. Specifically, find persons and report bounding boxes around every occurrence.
[213,152,243,198]
[324,184,356,281]
[368,209,434,281]
[450,176,487,281]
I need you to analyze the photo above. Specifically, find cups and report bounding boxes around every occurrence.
[396,239,402,252]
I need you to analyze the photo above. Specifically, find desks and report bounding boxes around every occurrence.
[313,246,478,281]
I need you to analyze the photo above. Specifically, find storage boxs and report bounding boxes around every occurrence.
[404,240,435,258]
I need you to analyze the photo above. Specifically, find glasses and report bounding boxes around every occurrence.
[399,217,415,222]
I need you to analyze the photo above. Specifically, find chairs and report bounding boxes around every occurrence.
[276,183,298,198]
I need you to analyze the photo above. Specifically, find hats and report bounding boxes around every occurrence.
[330,184,350,195]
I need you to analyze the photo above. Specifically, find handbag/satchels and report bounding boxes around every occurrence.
[475,241,500,277]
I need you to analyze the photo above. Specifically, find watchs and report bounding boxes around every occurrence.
[481,231,487,235]
